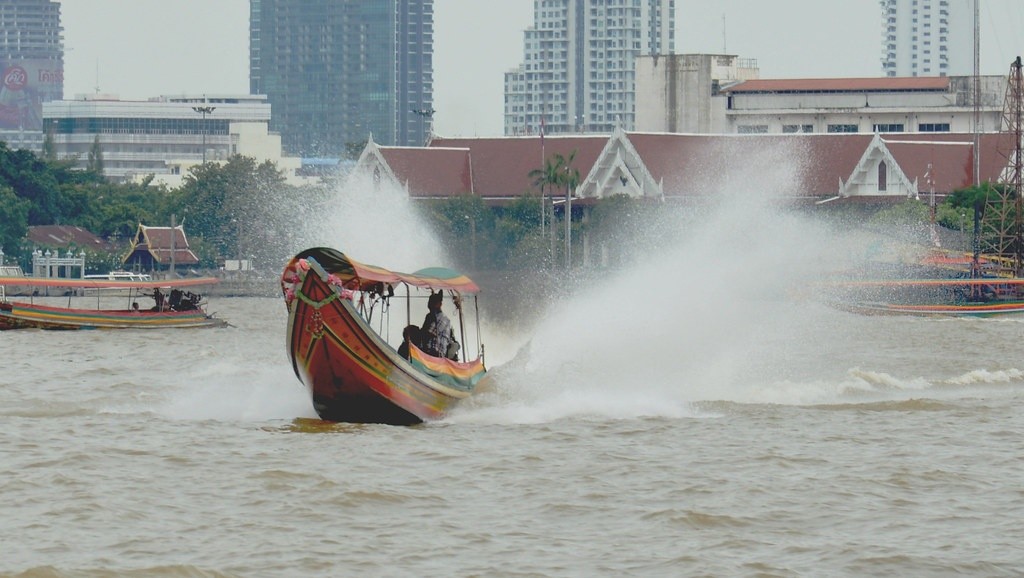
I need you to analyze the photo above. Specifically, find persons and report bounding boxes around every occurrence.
[397,325,420,363]
[144,288,166,305]
[446,329,461,361]
[418,294,449,358]
[132,302,140,314]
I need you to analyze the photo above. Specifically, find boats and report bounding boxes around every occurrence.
[279,246,489,435]
[0,275,229,332]
